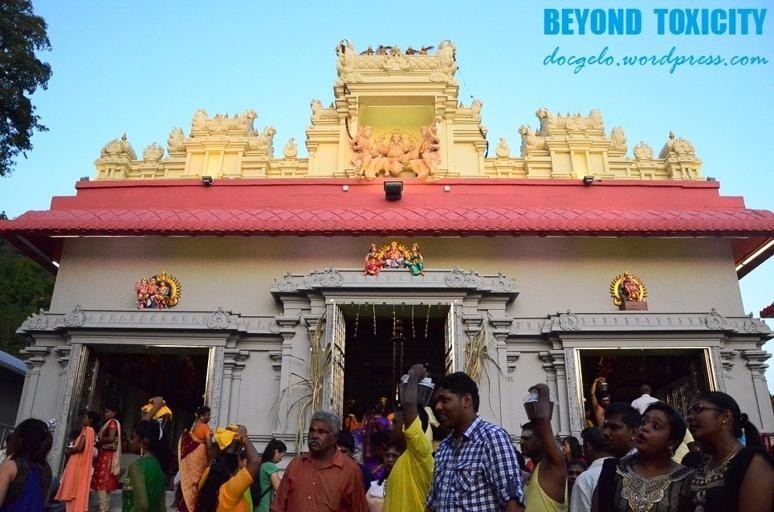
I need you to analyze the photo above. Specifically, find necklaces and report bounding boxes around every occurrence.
[704,444,740,484]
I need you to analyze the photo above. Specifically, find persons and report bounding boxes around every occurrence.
[1,417,54,512]
[333,431,355,460]
[383,363,434,512]
[254,440,287,512]
[143,397,172,471]
[170,405,213,512]
[383,240,406,268]
[363,244,383,274]
[590,402,697,512]
[560,435,581,462]
[122,420,168,512]
[622,275,640,300]
[351,125,440,178]
[134,276,170,308]
[571,427,614,512]
[570,406,643,512]
[365,431,410,511]
[196,424,262,512]
[686,391,774,512]
[564,461,585,487]
[632,382,661,413]
[428,372,526,512]
[590,376,612,428]
[95,402,121,511]
[520,383,567,512]
[271,411,368,512]
[55,410,99,512]
[403,243,424,275]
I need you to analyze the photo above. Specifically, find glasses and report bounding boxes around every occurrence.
[686,403,723,415]
